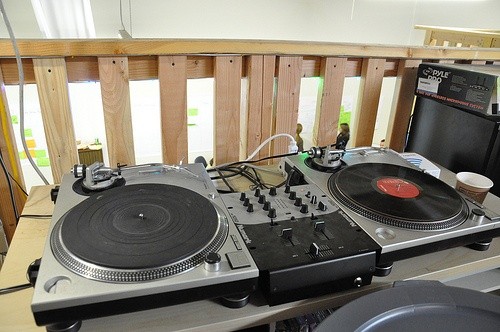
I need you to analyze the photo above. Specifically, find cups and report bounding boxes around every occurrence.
[456,172,494,203]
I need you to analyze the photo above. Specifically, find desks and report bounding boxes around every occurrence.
[0,162,500,332]
